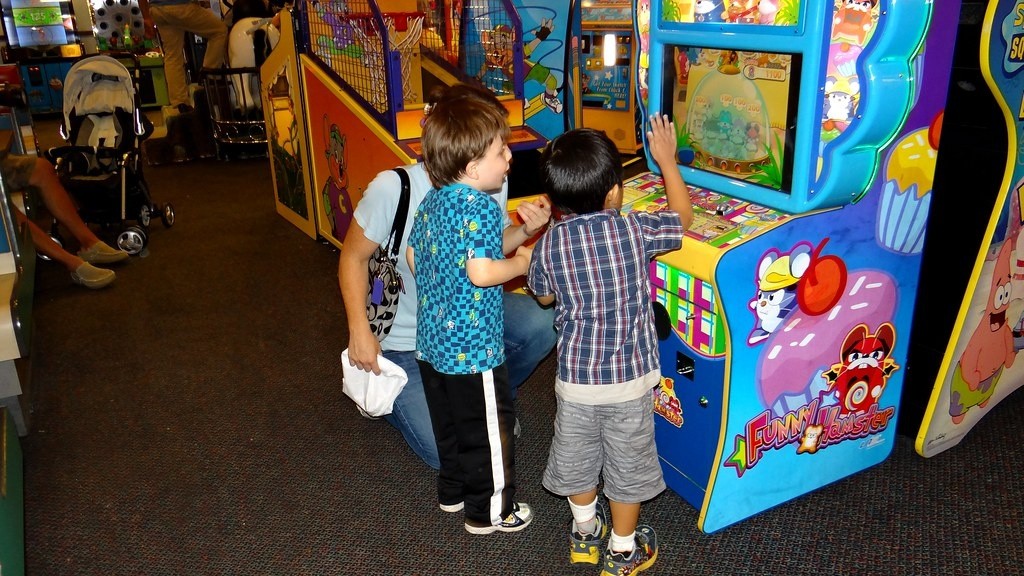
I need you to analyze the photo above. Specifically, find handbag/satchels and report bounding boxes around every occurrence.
[365,168,411,342]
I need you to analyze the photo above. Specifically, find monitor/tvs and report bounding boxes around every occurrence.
[661,44,802,195]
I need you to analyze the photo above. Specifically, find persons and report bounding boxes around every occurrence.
[231,0,297,64]
[525,111,695,576]
[402,93,552,535]
[338,81,561,471]
[148,0,228,113]
[0,150,129,288]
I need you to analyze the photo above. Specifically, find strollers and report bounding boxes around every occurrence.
[35,52,175,264]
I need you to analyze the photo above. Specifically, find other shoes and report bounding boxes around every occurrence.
[514,419,521,439]
[198,76,232,87]
[77,241,128,263]
[71,262,116,289]
[178,104,193,113]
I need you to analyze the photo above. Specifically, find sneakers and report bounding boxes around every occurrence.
[465,502,534,533]
[569,503,607,569]
[601,525,658,576]
[440,501,464,512]
